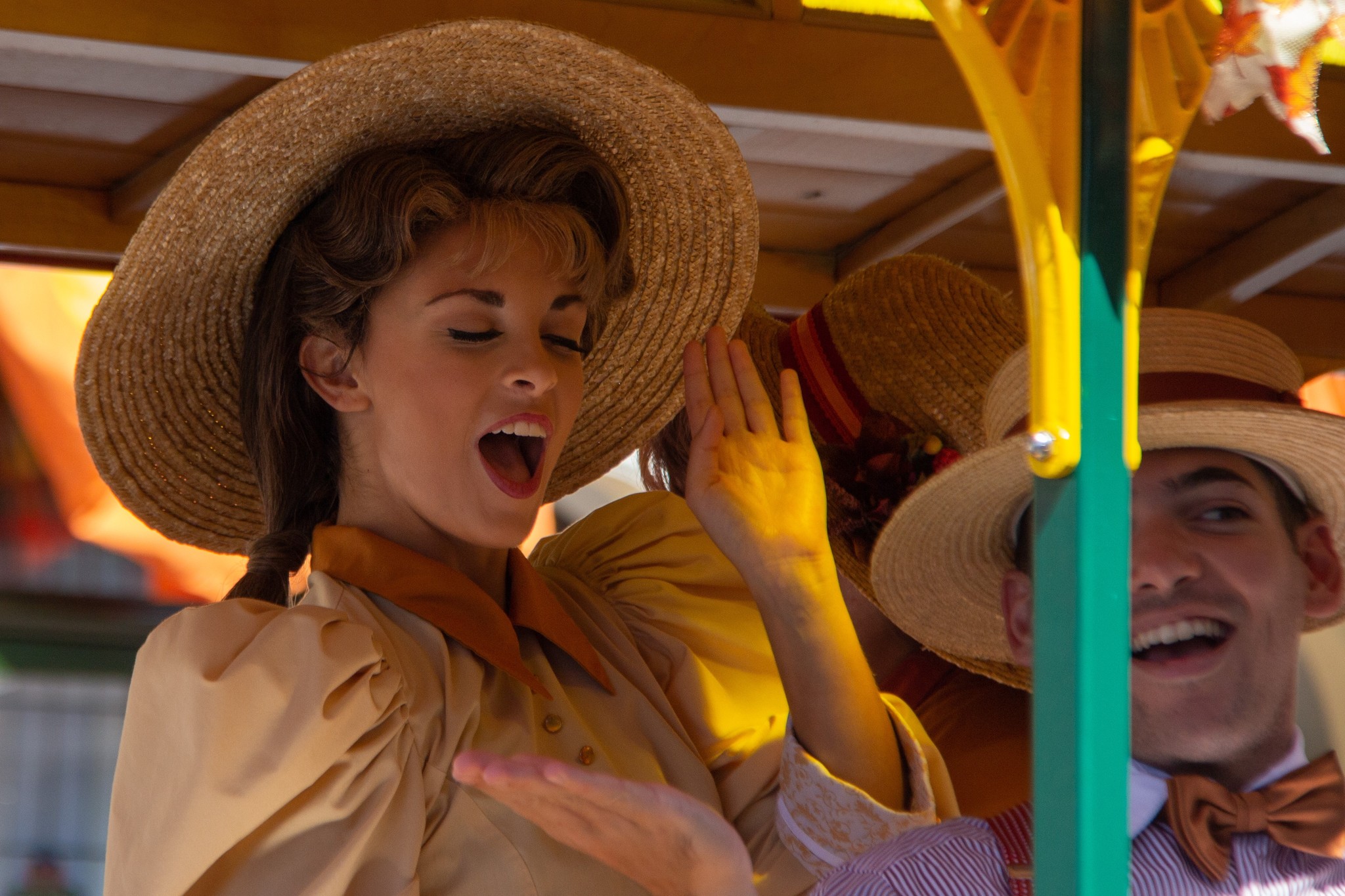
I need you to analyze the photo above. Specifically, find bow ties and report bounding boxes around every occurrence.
[1167,750,1345,883]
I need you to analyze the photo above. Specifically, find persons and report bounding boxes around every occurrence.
[638,250,1028,821]
[74,19,960,896]
[450,305,1345,896]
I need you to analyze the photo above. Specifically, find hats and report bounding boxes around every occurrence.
[870,307,1345,695]
[73,16,759,557]
[732,253,1033,621]
